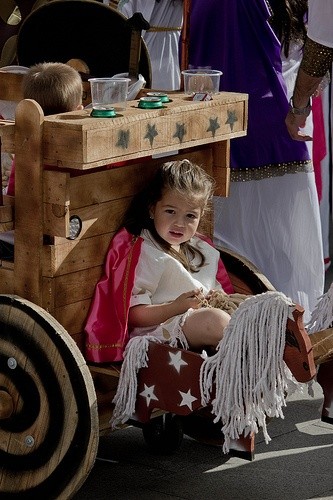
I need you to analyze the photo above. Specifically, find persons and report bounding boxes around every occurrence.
[285,0,333,142]
[101,0,183,91]
[85,159,234,363]
[178,0,325,330]
[0,62,83,263]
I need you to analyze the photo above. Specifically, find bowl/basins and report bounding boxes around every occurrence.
[88,78,131,110]
[182,69,223,96]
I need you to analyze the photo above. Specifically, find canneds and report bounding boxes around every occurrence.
[146,92,169,103]
[90,105,116,118]
[138,97,163,109]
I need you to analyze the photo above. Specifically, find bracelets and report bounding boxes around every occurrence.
[288,97,311,115]
